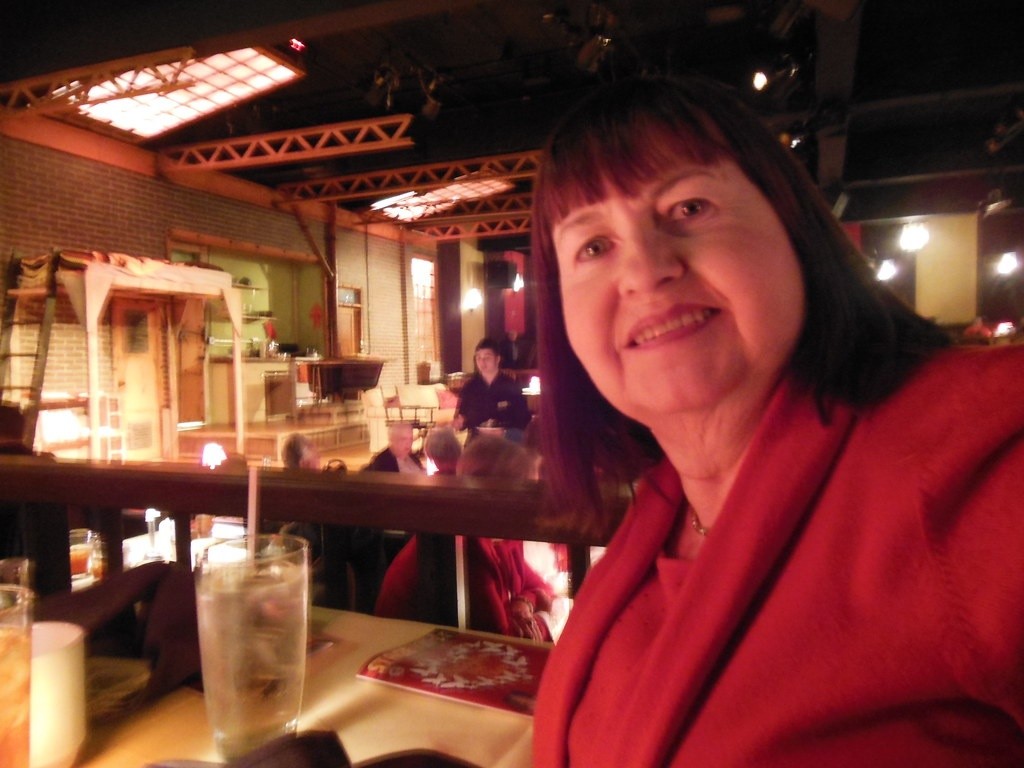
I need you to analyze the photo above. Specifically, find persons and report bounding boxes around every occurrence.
[528,70,1023,768]
[262,433,323,564]
[373,432,574,644]
[453,337,532,443]
[500,329,522,368]
[372,420,428,479]
[0,405,72,601]
[963,317,992,338]
[424,427,465,477]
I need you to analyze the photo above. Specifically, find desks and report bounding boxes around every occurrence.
[70,603,554,768]
[178,421,372,537]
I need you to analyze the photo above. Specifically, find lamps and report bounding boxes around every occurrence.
[746,57,803,97]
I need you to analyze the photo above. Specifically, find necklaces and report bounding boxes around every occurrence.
[691,509,714,534]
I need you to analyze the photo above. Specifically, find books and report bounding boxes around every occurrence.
[357,627,551,718]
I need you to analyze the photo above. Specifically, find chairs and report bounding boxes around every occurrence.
[379,385,438,459]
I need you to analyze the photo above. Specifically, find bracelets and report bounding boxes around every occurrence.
[510,597,533,612]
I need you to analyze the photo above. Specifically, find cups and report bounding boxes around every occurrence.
[196,535,307,762]
[0,585,36,768]
[30,622,84,768]
